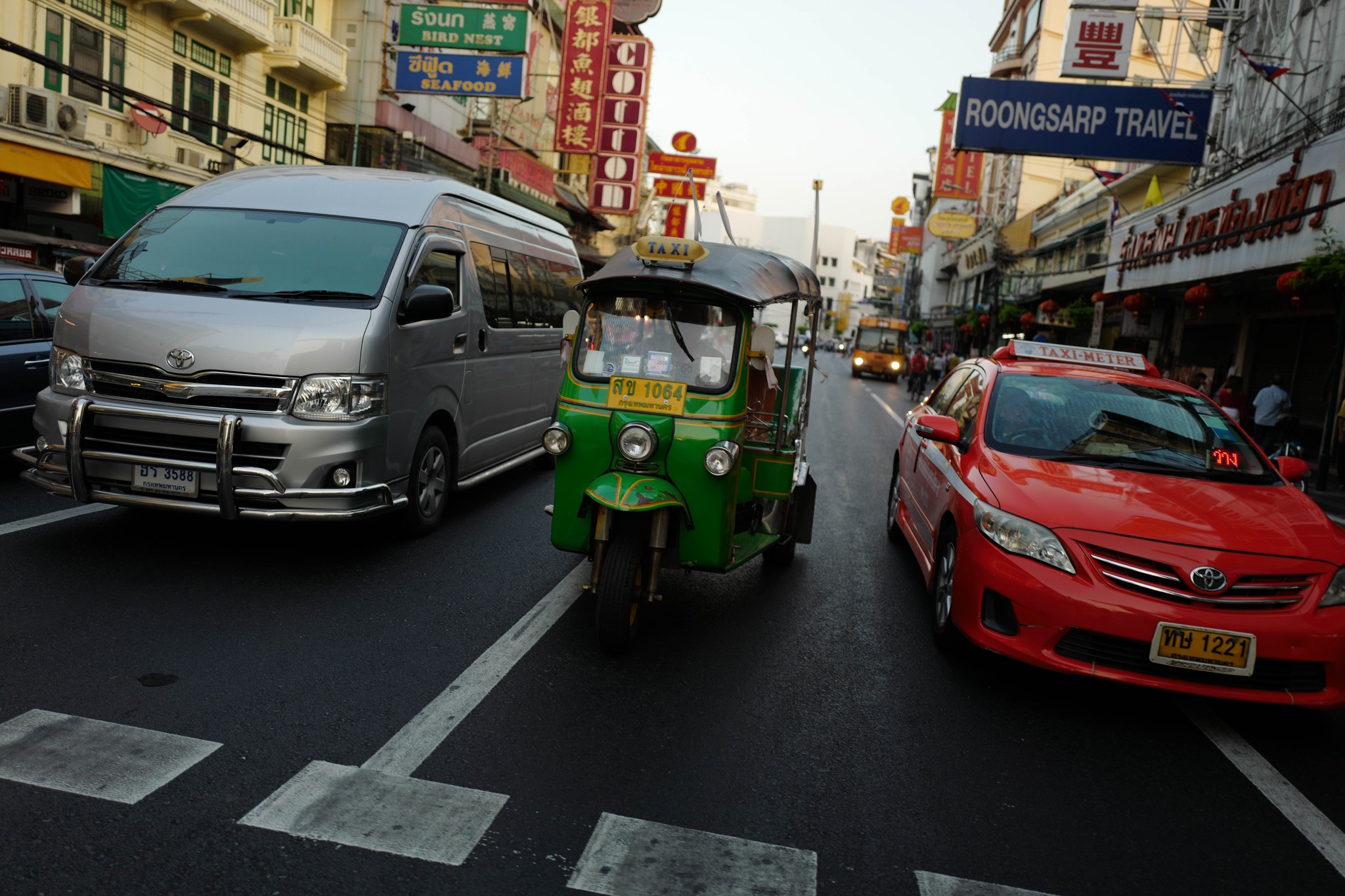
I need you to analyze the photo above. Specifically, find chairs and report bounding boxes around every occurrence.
[746,362,775,442]
[998,386,1032,438]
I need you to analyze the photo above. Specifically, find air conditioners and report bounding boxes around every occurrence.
[175,147,205,168]
[493,166,512,183]
[5,82,90,138]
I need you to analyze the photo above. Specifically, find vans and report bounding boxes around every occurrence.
[10,165,588,542]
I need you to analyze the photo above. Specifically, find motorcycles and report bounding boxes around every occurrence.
[541,235,824,634]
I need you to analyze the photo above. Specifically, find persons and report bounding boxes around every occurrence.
[805,338,852,358]
[629,298,726,385]
[903,346,964,394]
[995,387,1033,441]
[1161,355,1289,455]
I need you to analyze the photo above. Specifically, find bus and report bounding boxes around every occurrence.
[851,317,908,383]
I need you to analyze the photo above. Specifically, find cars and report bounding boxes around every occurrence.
[773,330,848,353]
[885,338,1345,724]
[0,256,77,454]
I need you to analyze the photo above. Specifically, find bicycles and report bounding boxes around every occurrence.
[910,367,928,404]
